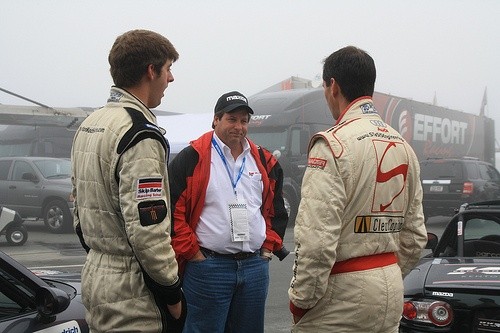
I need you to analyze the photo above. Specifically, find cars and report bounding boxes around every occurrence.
[397,200,500,333]
[0,249,91,333]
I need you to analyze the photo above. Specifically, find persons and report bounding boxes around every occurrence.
[287,46,428,333]
[168,91,289,333]
[71,30,187,333]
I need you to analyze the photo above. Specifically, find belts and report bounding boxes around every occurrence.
[200,247,258,260]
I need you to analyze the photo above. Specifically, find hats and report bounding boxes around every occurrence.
[214,91,254,114]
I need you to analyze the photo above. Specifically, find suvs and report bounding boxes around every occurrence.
[0,156,74,233]
[419,156,500,226]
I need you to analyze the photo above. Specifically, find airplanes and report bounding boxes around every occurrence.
[0,88,185,156]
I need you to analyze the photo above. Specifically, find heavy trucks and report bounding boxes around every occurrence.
[247,77,496,228]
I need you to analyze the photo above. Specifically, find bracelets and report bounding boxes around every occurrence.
[261,252,273,261]
